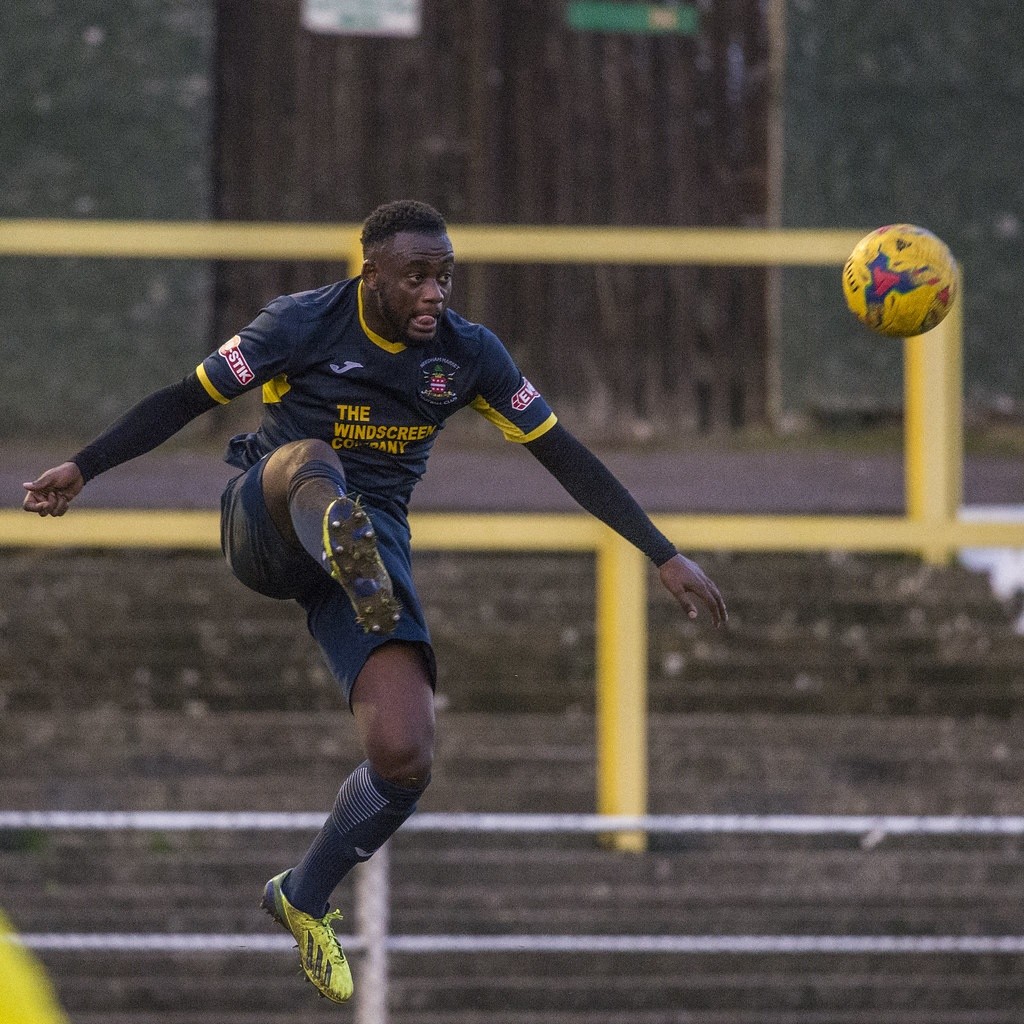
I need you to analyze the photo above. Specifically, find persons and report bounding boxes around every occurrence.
[22,202,731,1002]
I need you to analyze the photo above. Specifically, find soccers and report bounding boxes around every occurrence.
[841,222,960,338]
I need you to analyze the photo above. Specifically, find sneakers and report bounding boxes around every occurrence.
[322,496,401,633]
[260,870,357,1005]
